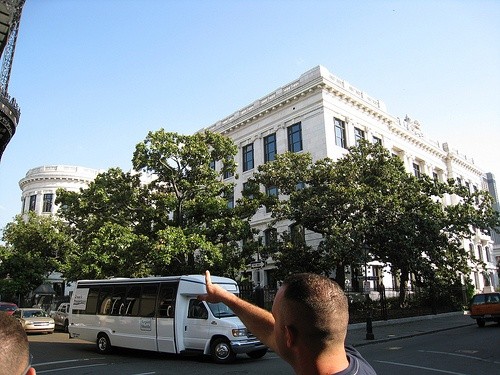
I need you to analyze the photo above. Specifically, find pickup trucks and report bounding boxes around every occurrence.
[50,303,70,333]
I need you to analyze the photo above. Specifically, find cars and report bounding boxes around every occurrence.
[0,301,18,316]
[12,308,55,335]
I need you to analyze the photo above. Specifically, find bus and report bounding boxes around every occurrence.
[68,275,269,364]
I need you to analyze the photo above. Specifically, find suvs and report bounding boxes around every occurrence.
[470,292,500,328]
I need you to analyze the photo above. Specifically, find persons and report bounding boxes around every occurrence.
[197,270,377,375]
[0,311,36,375]
